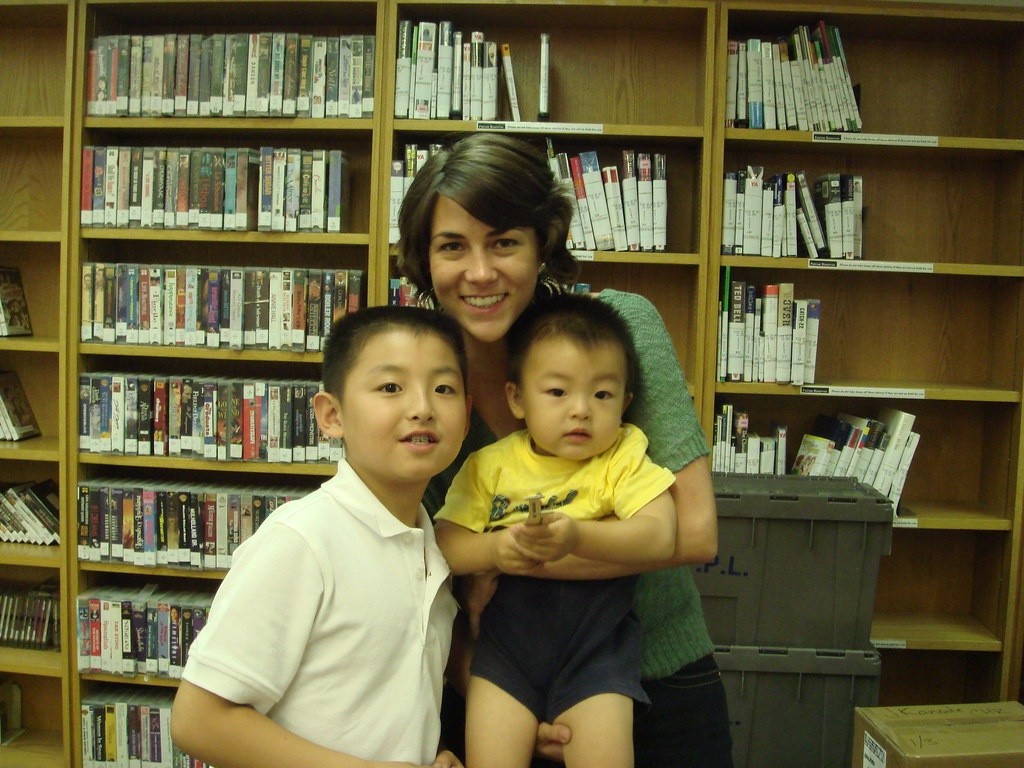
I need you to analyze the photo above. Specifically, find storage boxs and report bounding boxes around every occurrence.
[688,470,895,652]
[710,651,880,764]
[850,701,1023,768]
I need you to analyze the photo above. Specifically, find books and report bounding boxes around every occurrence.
[0,21,922,768]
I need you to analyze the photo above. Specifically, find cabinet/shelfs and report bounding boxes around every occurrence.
[0,1,1023,767]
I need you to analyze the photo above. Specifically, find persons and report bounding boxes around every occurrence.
[336,273,345,286]
[431,293,679,768]
[181,384,192,404]
[395,131,730,768]
[170,305,473,768]
